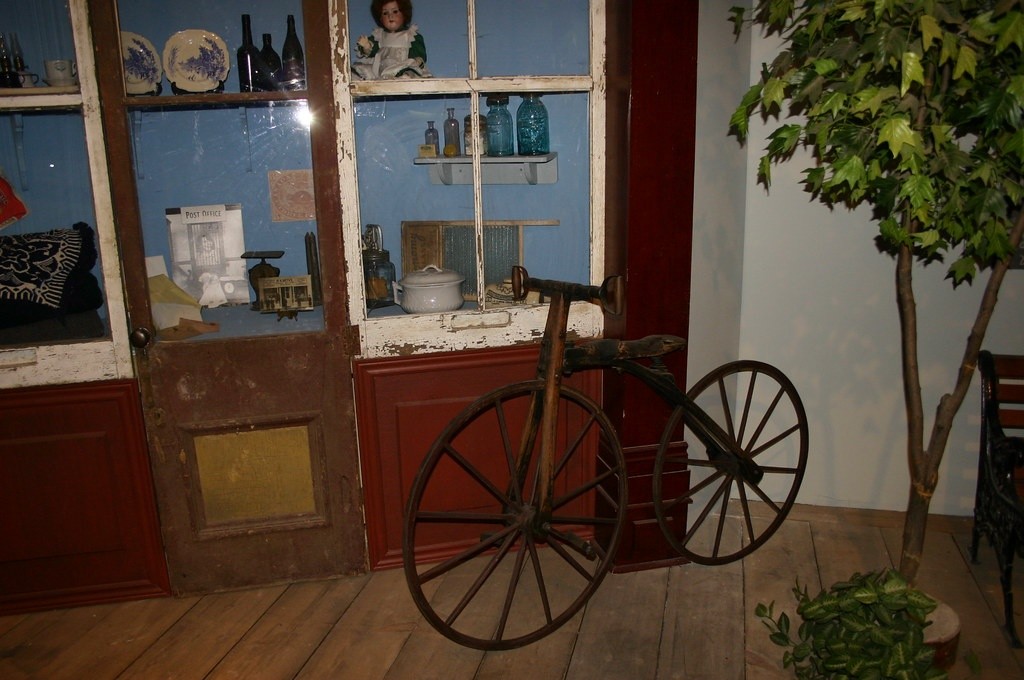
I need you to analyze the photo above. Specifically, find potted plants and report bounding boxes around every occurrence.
[725,0,1024,680]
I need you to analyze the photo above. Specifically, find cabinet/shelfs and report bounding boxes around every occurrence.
[0,0,699,613]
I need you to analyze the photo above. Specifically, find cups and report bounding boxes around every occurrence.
[43,59,78,78]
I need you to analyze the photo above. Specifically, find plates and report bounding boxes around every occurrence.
[43,76,76,87]
[120,31,163,94]
[162,28,231,91]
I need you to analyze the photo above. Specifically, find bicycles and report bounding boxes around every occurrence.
[402,263,810,650]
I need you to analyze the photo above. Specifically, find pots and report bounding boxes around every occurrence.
[391,264,466,313]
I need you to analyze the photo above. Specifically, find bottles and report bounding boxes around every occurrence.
[238,14,260,92]
[486,96,514,157]
[464,113,486,155]
[259,34,284,92]
[282,15,305,91]
[443,108,461,158]
[424,121,440,157]
[517,92,551,155]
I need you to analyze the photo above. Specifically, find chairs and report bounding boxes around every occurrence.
[969,350,1024,650]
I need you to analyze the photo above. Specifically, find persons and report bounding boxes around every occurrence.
[350,0,434,81]
[194,233,219,264]
